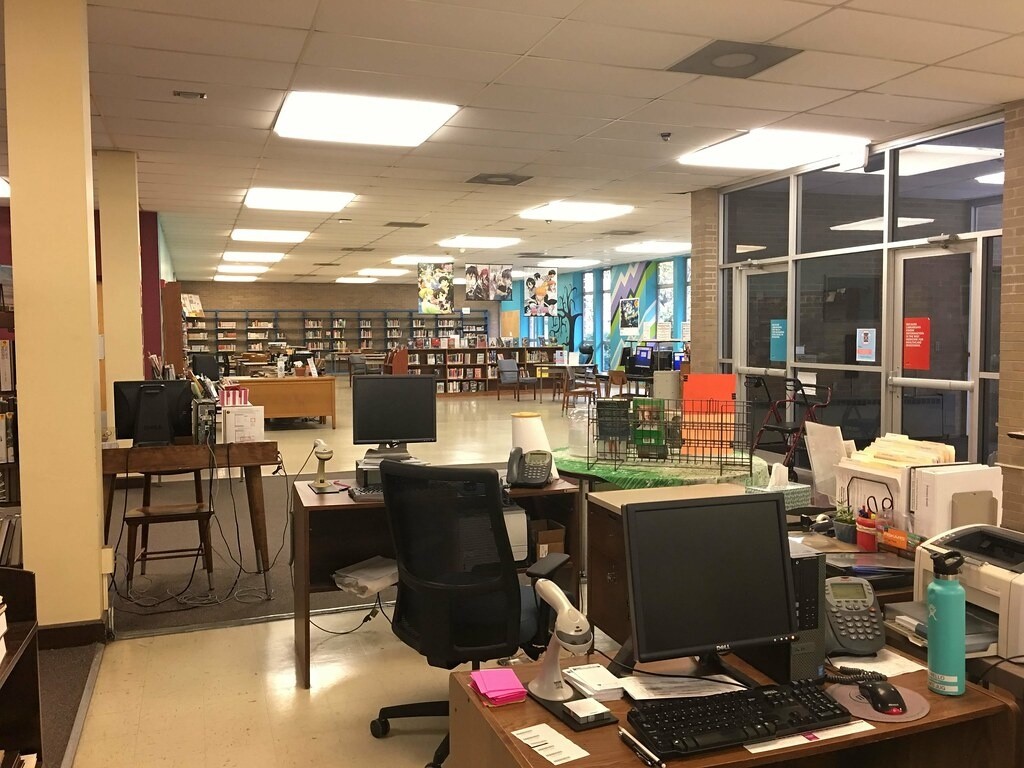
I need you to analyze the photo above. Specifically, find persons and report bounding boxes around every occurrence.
[636,411,659,430]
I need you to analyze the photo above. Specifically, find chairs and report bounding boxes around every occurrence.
[222,352,229,376]
[745,374,831,482]
[347,354,367,387]
[496,344,646,417]
[193,354,219,381]
[370,459,571,768]
[124,468,214,598]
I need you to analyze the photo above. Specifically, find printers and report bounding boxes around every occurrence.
[884,523,1024,664]
[452,499,528,571]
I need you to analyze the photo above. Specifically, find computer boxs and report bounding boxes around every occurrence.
[356,460,431,488]
[190,398,216,446]
[735,539,826,688]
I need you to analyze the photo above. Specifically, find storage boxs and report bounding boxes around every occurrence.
[527,519,565,563]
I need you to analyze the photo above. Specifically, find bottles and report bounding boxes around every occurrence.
[926,551,966,696]
[278,358,285,379]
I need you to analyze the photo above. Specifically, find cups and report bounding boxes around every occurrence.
[855,517,894,552]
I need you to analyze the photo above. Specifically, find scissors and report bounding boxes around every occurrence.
[867,495,893,515]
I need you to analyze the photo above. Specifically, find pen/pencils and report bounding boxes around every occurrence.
[857,505,872,518]
[617,724,666,768]
[617,731,656,767]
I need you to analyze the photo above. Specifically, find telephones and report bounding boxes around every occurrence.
[507,447,554,489]
[824,575,887,656]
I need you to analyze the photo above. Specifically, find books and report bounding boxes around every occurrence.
[185,319,372,355]
[0,595,38,768]
[0,264,22,569]
[385,318,558,394]
[595,394,682,448]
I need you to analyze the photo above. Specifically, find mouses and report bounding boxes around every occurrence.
[859,681,907,715]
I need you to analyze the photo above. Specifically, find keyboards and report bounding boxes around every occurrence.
[348,485,384,502]
[626,678,852,761]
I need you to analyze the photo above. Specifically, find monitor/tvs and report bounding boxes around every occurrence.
[622,345,690,378]
[352,375,437,465]
[622,491,800,678]
[288,353,314,368]
[114,379,193,447]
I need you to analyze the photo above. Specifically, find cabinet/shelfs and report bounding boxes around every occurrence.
[0,566,43,768]
[164,282,563,397]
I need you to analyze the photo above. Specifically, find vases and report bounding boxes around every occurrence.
[296,369,306,376]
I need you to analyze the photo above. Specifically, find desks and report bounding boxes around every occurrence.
[449,482,1024,768]
[553,446,767,583]
[294,462,580,689]
[533,364,594,404]
[624,339,691,372]
[101,442,277,594]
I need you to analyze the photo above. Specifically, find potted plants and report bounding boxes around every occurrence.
[832,498,856,543]
